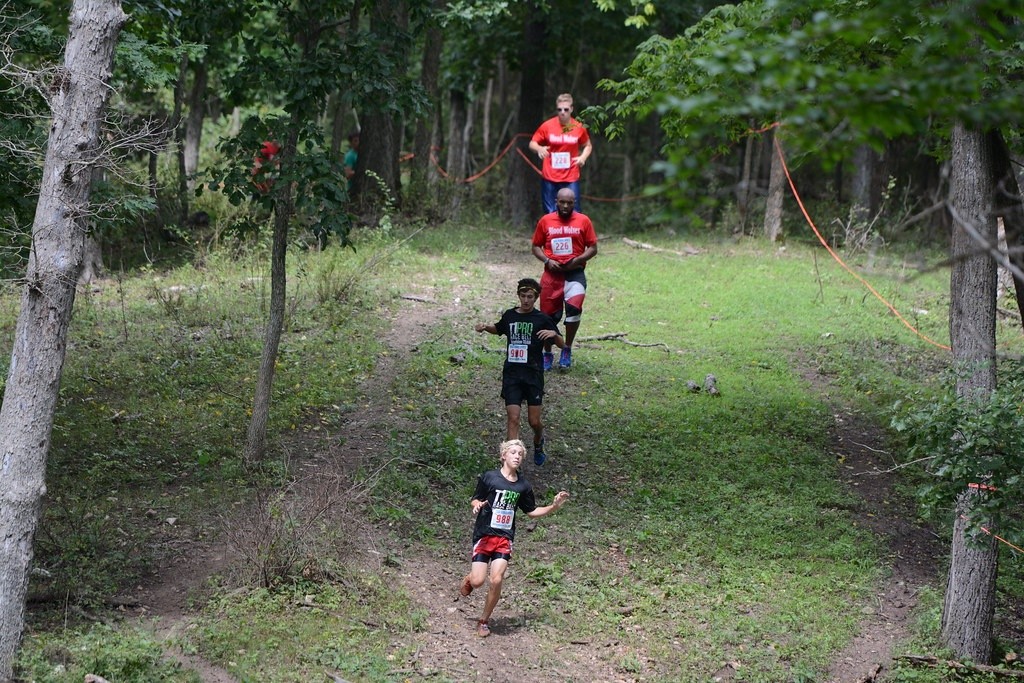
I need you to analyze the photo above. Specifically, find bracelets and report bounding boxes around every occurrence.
[543,257,550,268]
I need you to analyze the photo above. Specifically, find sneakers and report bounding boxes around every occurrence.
[559,348,571,370]
[478,619,490,637]
[460,573,473,597]
[544,352,554,371]
[533,435,545,466]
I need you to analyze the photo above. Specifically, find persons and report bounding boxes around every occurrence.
[475,278,565,466]
[529,93,593,216]
[531,187,597,372]
[461,439,569,638]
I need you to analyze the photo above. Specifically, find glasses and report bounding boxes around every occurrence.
[557,108,570,112]
